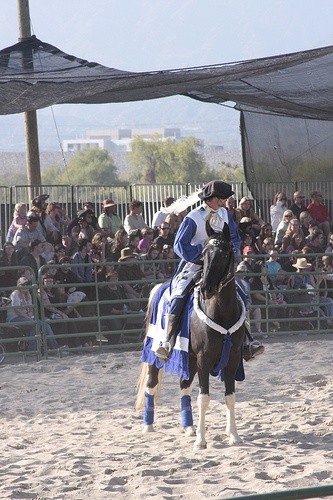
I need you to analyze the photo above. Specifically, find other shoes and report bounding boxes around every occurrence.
[96,334,109,343]
[244,345,265,362]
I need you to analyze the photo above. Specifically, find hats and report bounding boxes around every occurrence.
[77,210,89,218]
[198,180,235,201]
[103,199,115,208]
[243,246,254,254]
[29,239,46,249]
[17,277,30,288]
[291,258,312,269]
[119,248,137,261]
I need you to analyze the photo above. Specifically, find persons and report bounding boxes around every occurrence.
[154,180,266,362]
[0,189,333,350]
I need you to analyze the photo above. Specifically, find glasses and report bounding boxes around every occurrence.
[41,245,45,248]
[161,228,169,230]
[148,232,154,235]
[285,215,292,217]
[298,196,305,198]
[21,283,29,286]
[55,207,60,209]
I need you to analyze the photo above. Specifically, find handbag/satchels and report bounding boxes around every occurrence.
[156,347,168,364]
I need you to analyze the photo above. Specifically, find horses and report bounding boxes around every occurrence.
[135,220,251,450]
